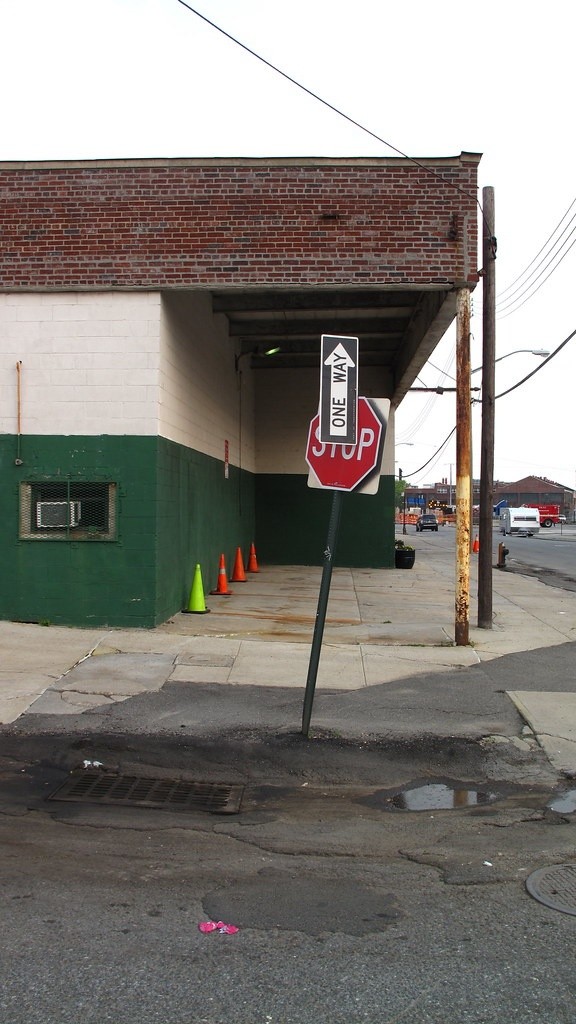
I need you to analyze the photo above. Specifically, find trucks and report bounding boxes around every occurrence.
[519,501,561,528]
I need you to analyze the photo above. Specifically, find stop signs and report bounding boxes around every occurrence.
[306,395,390,492]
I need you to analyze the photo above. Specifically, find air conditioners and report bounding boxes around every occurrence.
[37,501,81,528]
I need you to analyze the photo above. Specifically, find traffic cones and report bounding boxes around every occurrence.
[245,544,264,575]
[211,555,235,597]
[227,548,251,584]
[180,561,211,615]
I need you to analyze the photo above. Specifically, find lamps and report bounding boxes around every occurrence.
[235,343,280,372]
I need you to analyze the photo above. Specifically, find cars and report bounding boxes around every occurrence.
[416,512,441,532]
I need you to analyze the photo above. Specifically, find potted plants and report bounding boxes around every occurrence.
[395,545,416,569]
